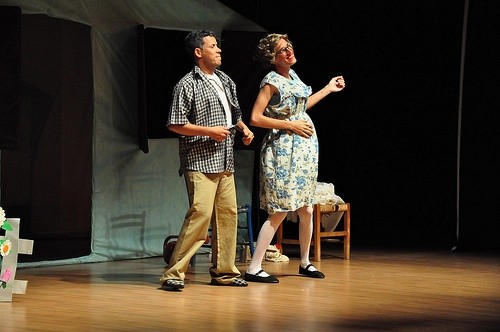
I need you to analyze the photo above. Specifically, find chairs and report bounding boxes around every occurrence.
[275,182,350,262]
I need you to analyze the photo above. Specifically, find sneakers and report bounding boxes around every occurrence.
[211,277,249,286]
[162,279,185,289]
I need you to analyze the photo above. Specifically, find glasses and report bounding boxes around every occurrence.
[275,41,292,56]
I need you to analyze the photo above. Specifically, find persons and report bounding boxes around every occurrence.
[245,35,345,282]
[159,30,255,291]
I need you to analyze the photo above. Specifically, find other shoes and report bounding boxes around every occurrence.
[299,264,325,278]
[243,269,279,283]
